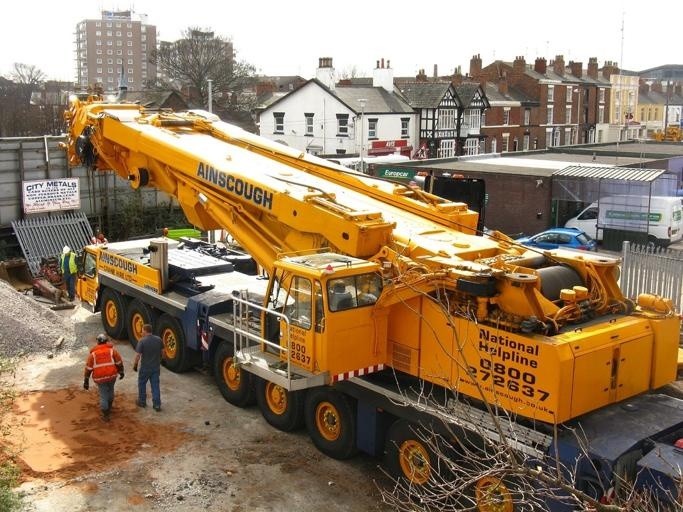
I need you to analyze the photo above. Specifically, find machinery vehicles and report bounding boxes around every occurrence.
[58,97,682,510]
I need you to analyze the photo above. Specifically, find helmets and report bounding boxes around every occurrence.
[63,246,71,254]
[97,334,108,344]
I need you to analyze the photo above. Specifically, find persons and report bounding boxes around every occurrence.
[59,246,79,302]
[98,233,108,243]
[133,323,164,412]
[83,333,125,421]
[89,237,101,245]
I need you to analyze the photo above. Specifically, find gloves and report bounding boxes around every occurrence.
[120,373,124,379]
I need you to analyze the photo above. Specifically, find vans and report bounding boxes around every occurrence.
[564,193,682,250]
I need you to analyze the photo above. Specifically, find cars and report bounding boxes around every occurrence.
[512,227,597,255]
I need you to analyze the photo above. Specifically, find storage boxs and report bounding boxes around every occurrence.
[165,229,202,239]
